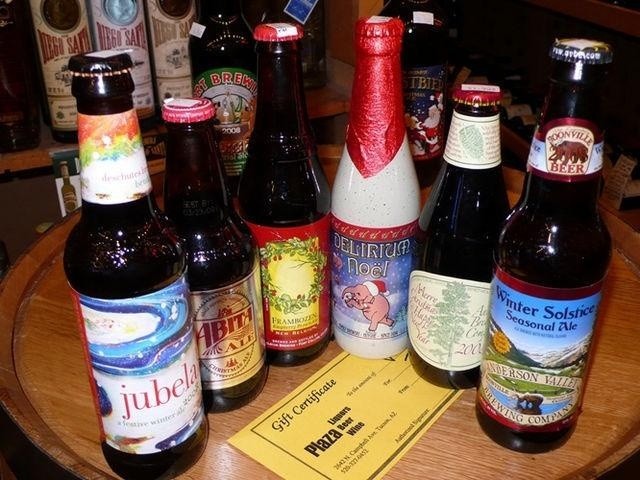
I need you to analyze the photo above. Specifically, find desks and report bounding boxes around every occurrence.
[0,140,639,478]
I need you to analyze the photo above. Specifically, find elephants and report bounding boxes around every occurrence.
[548,140,589,166]
[515,392,543,409]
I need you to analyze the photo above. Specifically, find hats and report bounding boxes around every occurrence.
[363,280,388,297]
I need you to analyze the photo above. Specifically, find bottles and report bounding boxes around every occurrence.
[181,93,269,416]
[1,0,98,151]
[87,0,157,135]
[473,35,613,455]
[409,82,511,390]
[378,0,450,192]
[250,19,334,368]
[144,0,199,120]
[331,16,417,364]
[187,0,259,183]
[275,0,328,93]
[64,48,211,480]
